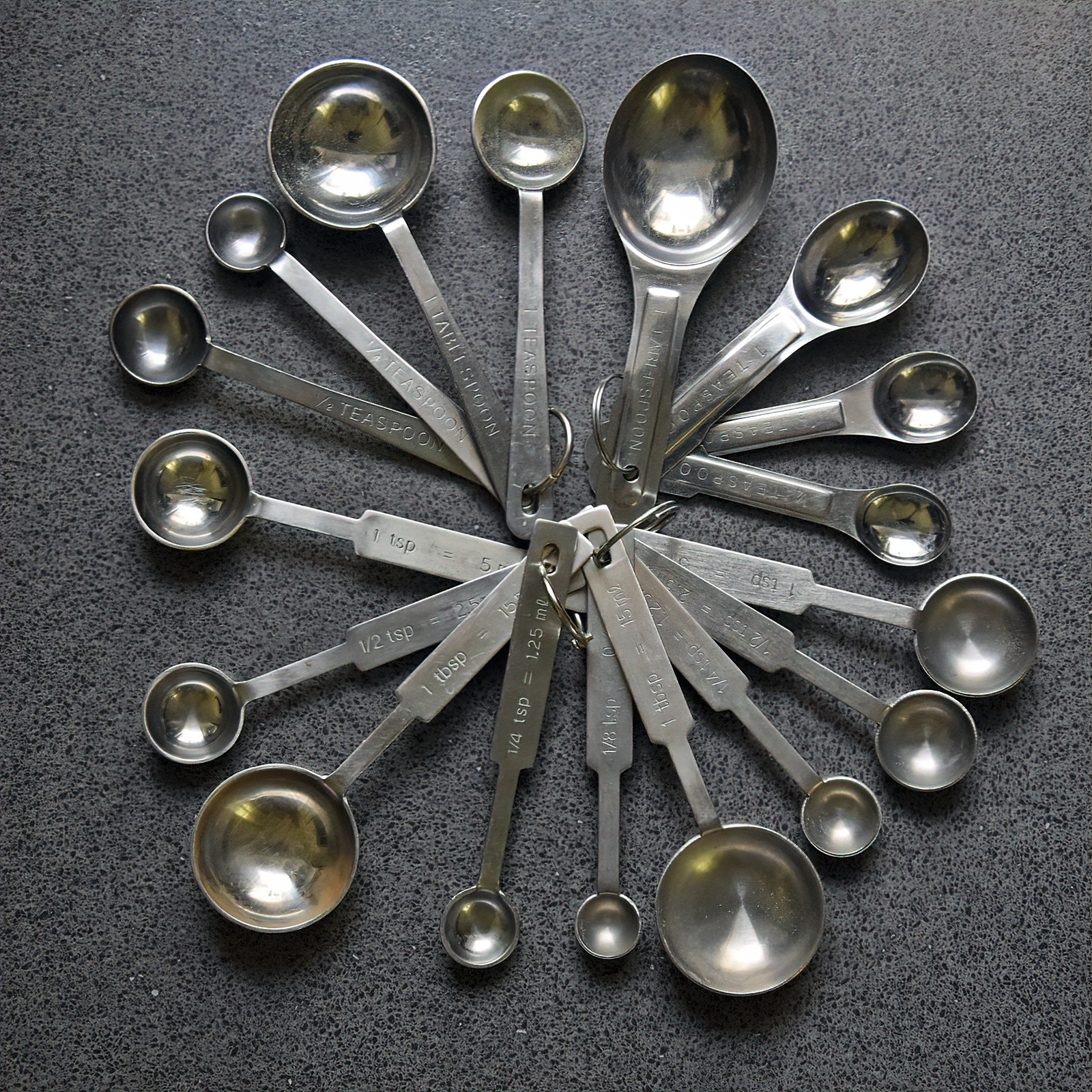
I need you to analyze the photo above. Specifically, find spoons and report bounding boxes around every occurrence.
[206,192,499,500]
[129,429,526,583]
[266,58,539,514]
[573,198,931,494]
[565,502,824,995]
[438,518,577,968]
[583,351,979,459]
[107,284,483,484]
[141,551,586,764]
[636,537,979,793]
[189,521,597,934]
[592,50,779,524]
[577,505,883,857]
[574,585,644,961]
[655,442,954,568]
[471,69,588,539]
[587,524,1040,697]
